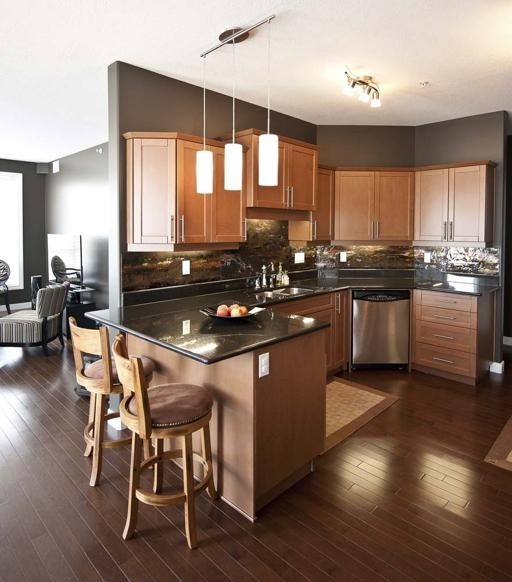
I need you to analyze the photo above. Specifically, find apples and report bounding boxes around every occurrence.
[217,303,247,316]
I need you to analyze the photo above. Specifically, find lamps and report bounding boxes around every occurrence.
[182,320,190,336]
[196,13,279,193]
[342,72,381,108]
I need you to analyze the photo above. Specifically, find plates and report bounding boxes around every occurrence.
[199,301,267,325]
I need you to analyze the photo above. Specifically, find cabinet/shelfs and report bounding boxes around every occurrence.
[286,161,498,249]
[215,127,319,222]
[121,129,248,252]
[266,289,494,386]
[69,284,96,329]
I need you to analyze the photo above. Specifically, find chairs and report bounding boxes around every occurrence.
[111,333,220,549]
[0,281,70,351]
[51,256,81,284]
[0,260,11,314]
[69,317,156,488]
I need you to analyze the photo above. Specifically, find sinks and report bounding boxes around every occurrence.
[252,290,284,299]
[273,287,314,295]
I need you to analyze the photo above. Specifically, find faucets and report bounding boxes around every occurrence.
[261,265,266,287]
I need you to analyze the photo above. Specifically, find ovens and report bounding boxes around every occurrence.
[351,289,410,365]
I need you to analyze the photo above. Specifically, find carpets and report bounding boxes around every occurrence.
[319,374,401,456]
[483,416,512,472]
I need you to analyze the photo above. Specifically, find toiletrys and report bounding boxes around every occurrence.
[275,260,284,288]
[269,262,276,283]
